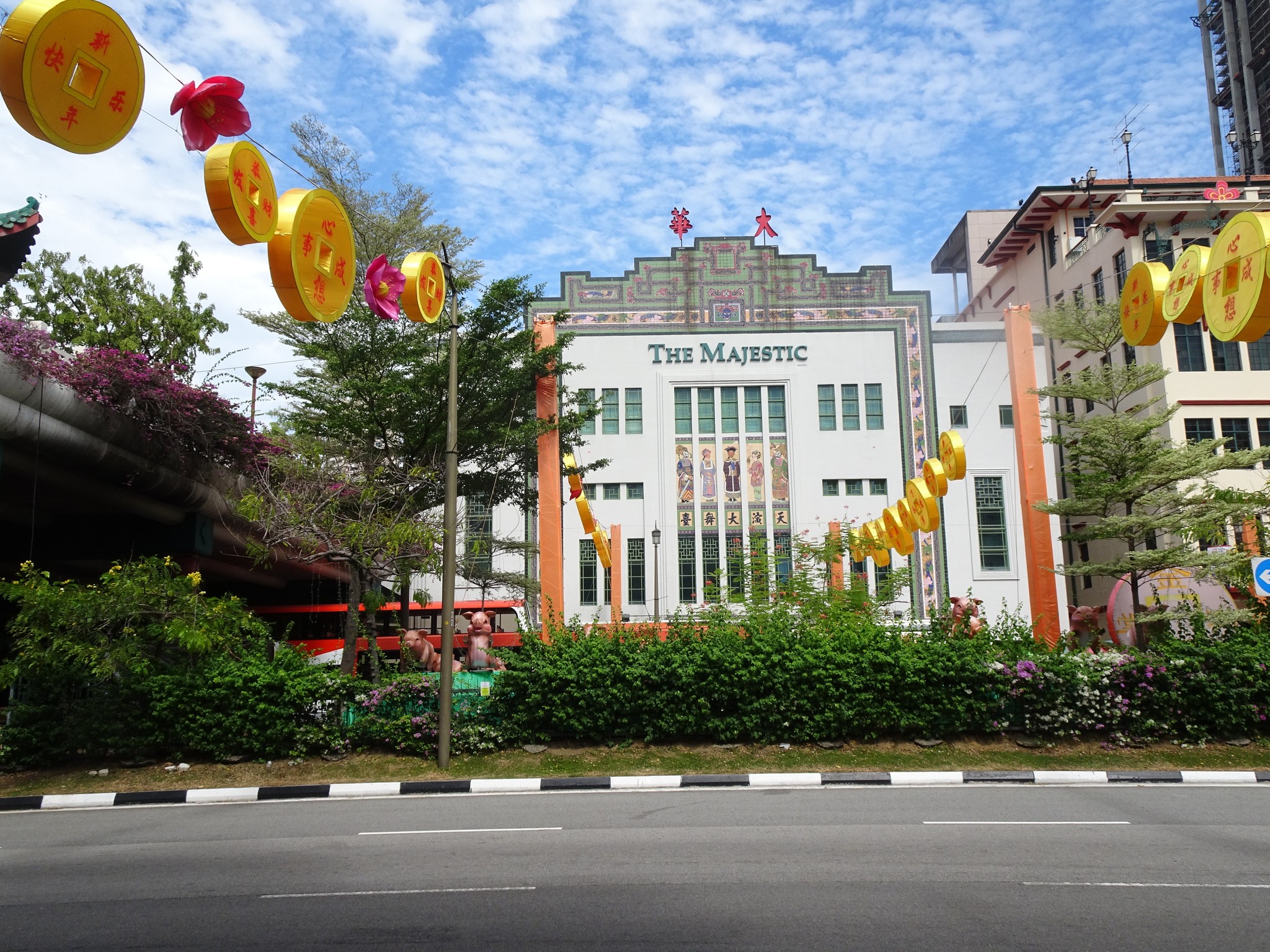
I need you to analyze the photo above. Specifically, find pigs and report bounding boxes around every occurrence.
[937,595,989,642]
[1125,602,1170,653]
[394,627,468,676]
[574,624,608,638]
[1065,604,1106,655]
[461,609,507,674]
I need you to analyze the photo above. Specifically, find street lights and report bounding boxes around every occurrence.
[1225,128,1262,187]
[1077,166,1098,225]
[1121,130,1134,189]
[243,367,267,435]
[651,520,660,623]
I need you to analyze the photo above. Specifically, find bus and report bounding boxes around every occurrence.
[241,598,538,706]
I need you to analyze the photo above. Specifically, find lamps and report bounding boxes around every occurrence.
[894,610,902,620]
[1077,166,1096,193]
[1120,130,1133,143]
[621,614,630,623]
[1018,199,1023,210]
[1071,178,1077,189]
[1054,235,1059,242]
[987,239,991,247]
[1223,129,1262,150]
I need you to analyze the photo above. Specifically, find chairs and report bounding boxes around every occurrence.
[457,628,461,634]
[376,629,380,636]
[497,625,505,633]
[327,629,336,637]
[436,630,439,634]
